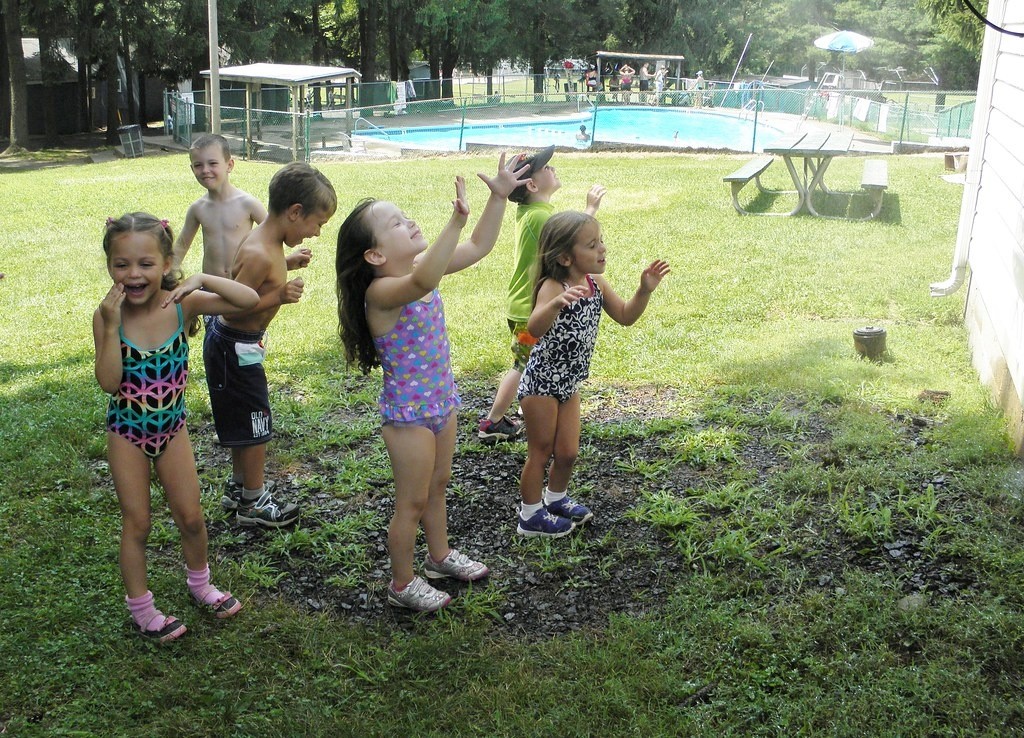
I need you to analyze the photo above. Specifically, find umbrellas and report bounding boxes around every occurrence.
[814,30,874,77]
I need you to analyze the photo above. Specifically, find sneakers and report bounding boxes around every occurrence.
[542,494,594,525]
[425,549,488,580]
[237,491,300,526]
[517,508,576,538]
[388,577,450,611]
[222,481,275,510]
[477,415,522,439]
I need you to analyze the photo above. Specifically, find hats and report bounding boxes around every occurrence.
[505,145,554,201]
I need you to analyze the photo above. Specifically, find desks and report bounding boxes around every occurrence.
[763,131,861,222]
[607,84,639,93]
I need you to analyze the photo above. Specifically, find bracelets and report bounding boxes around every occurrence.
[586,276,595,293]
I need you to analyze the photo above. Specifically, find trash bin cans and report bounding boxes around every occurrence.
[935,93,946,113]
[117,124,144,158]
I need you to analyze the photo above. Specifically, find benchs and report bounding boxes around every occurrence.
[722,155,774,215]
[862,158,890,221]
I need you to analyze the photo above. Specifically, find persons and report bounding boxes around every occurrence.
[692,71,705,110]
[335,152,532,609]
[619,64,636,107]
[517,211,670,538]
[576,125,589,142]
[609,76,619,103]
[328,90,336,110]
[93,212,261,641]
[587,66,597,87]
[172,134,268,326]
[494,91,502,102]
[307,90,312,109]
[638,63,656,105]
[655,65,668,91]
[554,73,560,92]
[477,146,605,442]
[204,163,336,527]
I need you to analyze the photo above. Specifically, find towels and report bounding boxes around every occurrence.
[852,98,872,121]
[387,80,417,109]
[877,103,890,132]
[825,92,842,120]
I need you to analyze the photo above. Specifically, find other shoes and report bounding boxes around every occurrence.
[133,610,186,642]
[189,584,242,618]
